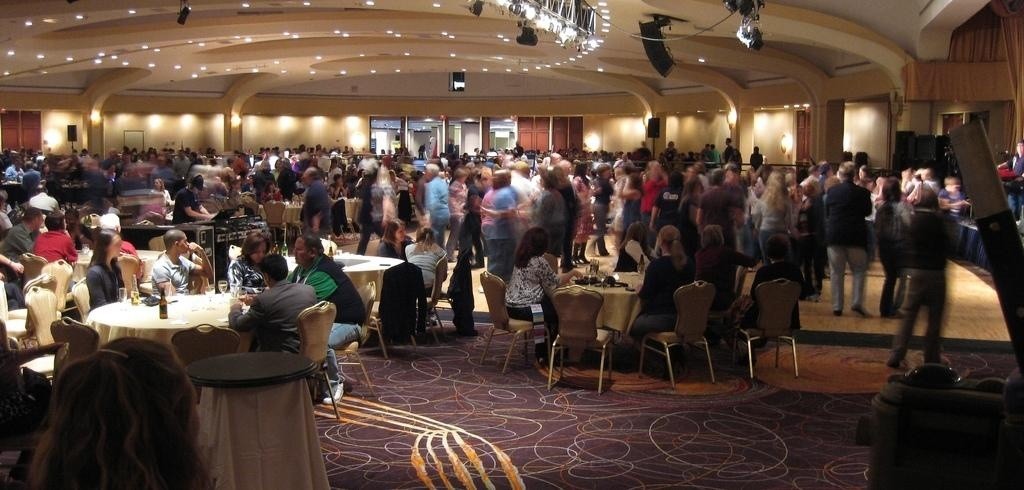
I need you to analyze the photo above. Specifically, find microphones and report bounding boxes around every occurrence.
[1000,150,1010,155]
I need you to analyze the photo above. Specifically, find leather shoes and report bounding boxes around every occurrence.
[448,260,456,262]
[472,263,478,268]
[852,305,871,318]
[834,310,841,315]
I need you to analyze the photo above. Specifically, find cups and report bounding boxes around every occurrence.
[335,250,342,256]
[119,288,127,305]
[589,258,598,275]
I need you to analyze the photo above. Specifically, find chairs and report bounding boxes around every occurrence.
[1,161,449,489]
[639,281,715,389]
[543,254,559,276]
[479,270,552,374]
[733,279,799,387]
[547,285,613,397]
[706,266,749,353]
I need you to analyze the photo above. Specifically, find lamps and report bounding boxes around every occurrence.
[725,1,764,52]
[725,108,737,128]
[462,1,489,16]
[498,0,601,55]
[779,134,792,156]
[177,1,190,26]
[641,111,651,131]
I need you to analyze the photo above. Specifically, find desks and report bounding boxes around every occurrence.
[555,266,648,365]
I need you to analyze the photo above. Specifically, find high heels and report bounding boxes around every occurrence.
[572,254,582,264]
[578,255,590,264]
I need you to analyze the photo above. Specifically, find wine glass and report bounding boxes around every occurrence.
[204,286,214,308]
[217,280,228,297]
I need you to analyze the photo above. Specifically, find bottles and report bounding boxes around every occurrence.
[129,273,138,304]
[328,247,333,261]
[273,244,288,257]
[158,286,167,319]
[638,254,645,275]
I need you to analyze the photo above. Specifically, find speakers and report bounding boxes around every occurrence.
[648,118,660,137]
[67,125,78,141]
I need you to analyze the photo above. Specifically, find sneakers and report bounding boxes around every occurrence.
[323,382,343,405]
